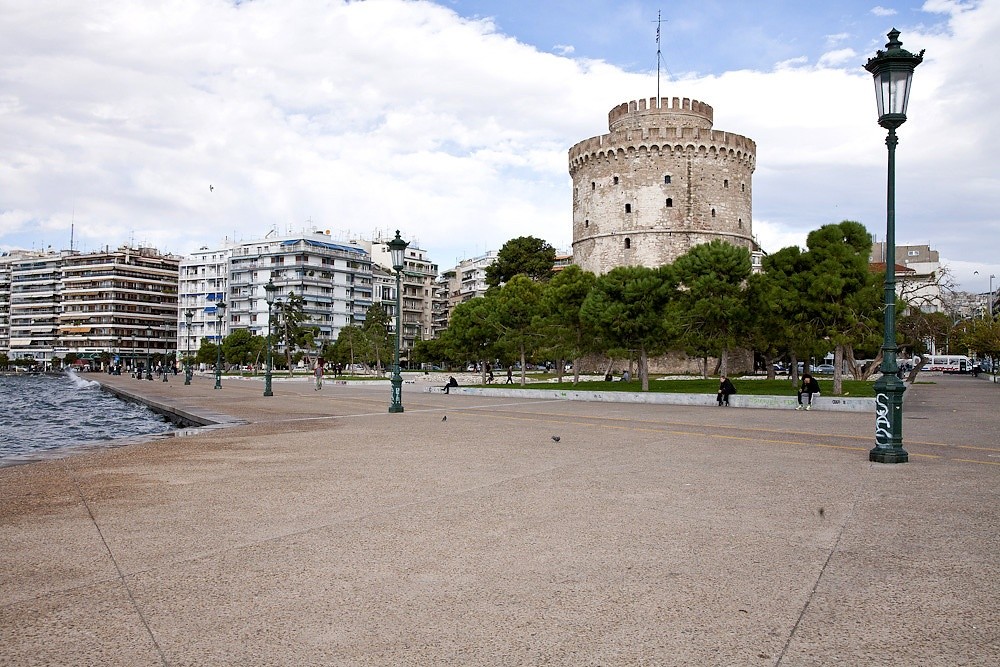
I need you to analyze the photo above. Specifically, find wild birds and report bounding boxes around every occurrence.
[209,184,213,192]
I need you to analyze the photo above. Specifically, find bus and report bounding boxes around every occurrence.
[921,354,978,374]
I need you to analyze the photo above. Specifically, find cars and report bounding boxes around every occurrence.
[465,359,575,374]
[292,364,309,372]
[753,358,1000,375]
[10,364,65,372]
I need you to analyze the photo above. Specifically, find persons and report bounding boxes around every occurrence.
[620,370,629,382]
[605,370,613,381]
[716,376,737,407]
[441,376,458,394]
[125,363,178,381]
[314,363,324,391]
[795,374,821,411]
[506,366,514,384]
[901,363,906,373]
[485,371,494,385]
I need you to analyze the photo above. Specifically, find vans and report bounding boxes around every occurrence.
[345,364,363,371]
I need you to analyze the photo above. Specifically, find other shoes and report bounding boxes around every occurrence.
[719,402,723,406]
[795,405,803,411]
[725,402,729,406]
[444,392,449,394]
[441,389,445,391]
[806,406,812,411]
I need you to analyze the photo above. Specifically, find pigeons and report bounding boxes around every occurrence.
[442,415,447,422]
[550,435,561,443]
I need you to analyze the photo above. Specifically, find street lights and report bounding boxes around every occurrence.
[384,229,411,413]
[184,309,194,386]
[108,339,113,361]
[162,318,171,382]
[263,278,279,397]
[117,335,122,374]
[862,25,926,465]
[214,298,227,390]
[130,331,137,379]
[989,274,996,315]
[144,323,153,380]
